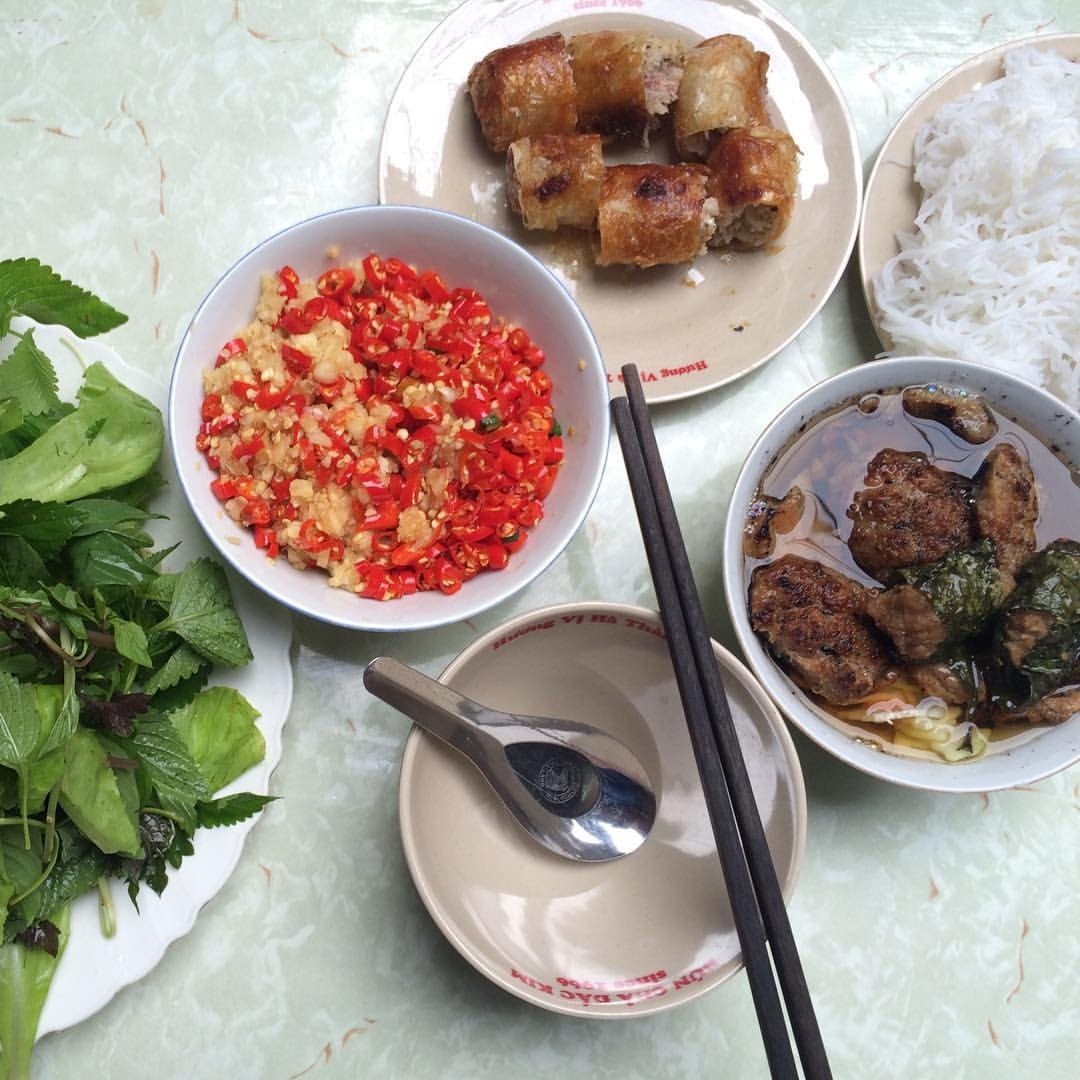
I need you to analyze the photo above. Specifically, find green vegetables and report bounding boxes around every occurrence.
[1,256,282,1080]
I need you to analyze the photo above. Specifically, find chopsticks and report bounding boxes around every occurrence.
[612,363,835,1079]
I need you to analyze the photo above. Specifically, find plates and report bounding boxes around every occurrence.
[858,35,1077,358]
[378,0,862,404]
[0,312,300,1044]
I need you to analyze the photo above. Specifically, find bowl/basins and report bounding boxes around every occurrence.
[397,601,805,1017]
[168,205,610,631]
[721,357,1080,796]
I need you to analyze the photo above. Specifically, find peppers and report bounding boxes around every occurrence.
[197,244,568,606]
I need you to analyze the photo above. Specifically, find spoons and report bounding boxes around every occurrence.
[357,656,656,863]
